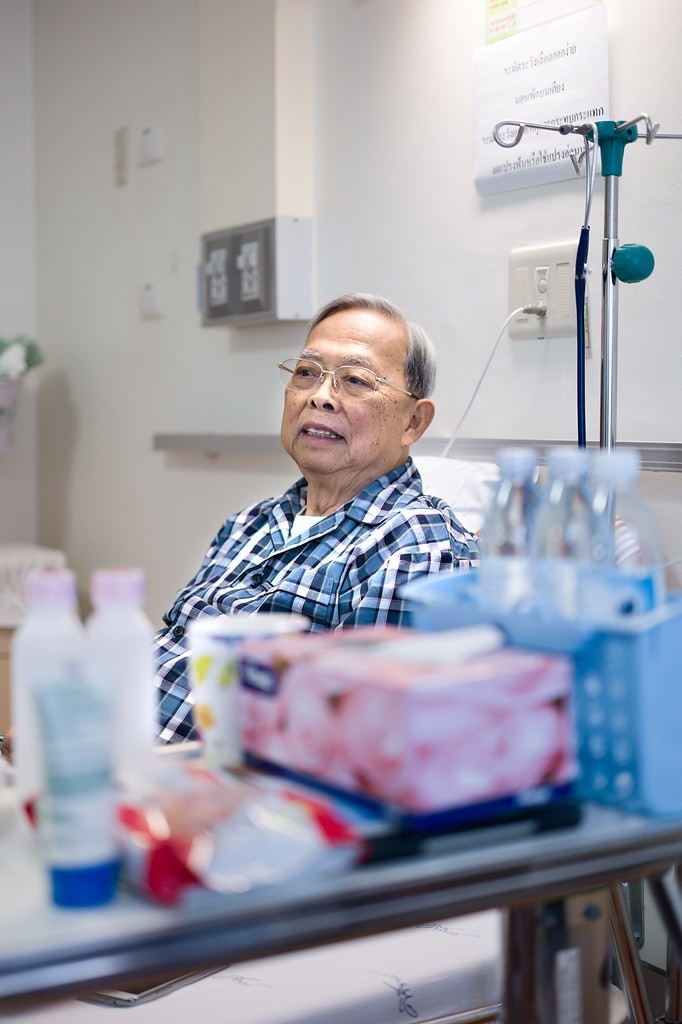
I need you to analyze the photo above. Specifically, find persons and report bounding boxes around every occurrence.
[0,292,482,769]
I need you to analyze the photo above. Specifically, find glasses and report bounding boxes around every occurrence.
[276,357,422,403]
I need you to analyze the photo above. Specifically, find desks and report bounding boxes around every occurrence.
[0,763,682,1024]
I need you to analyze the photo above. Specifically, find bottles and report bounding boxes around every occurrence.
[17,574,82,796]
[593,452,665,621]
[85,572,162,778]
[480,447,528,602]
[532,450,594,613]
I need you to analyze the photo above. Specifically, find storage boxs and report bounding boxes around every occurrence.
[399,556,682,823]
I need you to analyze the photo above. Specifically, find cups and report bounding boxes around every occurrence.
[185,612,310,765]
